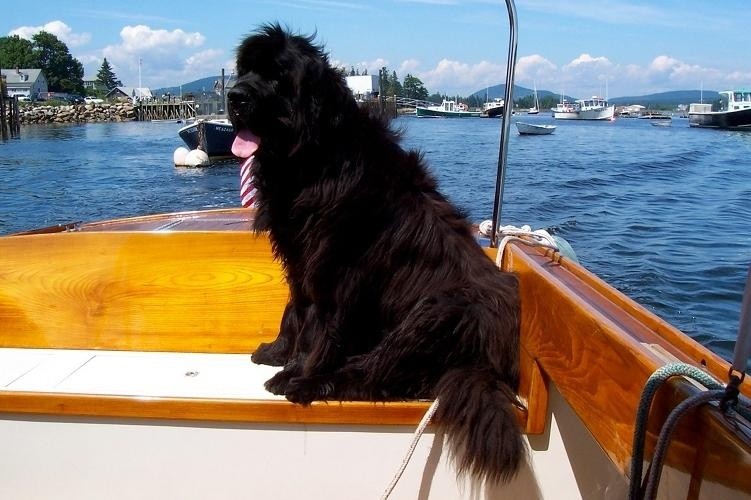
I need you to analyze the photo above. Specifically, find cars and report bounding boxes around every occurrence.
[9,92,104,105]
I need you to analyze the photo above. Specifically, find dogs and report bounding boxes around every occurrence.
[225,19,532,487]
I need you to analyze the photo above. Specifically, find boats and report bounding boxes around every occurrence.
[416,99,483,118]
[516,122,558,135]
[550,94,673,126]
[687,89,751,128]
[0,179,751,500]
[178,68,235,163]
[527,106,539,114]
[484,96,505,118]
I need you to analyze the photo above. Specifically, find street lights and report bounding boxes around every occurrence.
[138,56,143,101]
